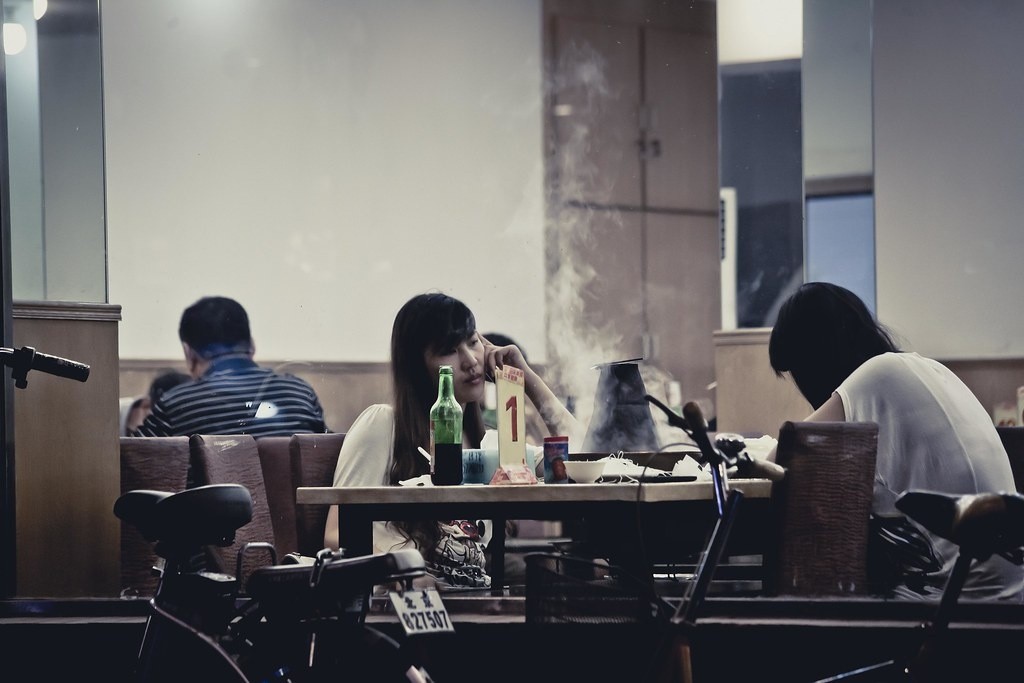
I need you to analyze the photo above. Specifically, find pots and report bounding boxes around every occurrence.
[567,363,707,482]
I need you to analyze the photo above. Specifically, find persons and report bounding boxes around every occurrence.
[324,293,579,592]
[768,282,1024,603]
[119,295,333,438]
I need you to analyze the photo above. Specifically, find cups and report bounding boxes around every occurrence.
[543,436,568,484]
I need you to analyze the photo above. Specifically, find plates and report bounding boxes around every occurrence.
[568,475,697,482]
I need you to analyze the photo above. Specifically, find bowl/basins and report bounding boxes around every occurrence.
[461,449,536,484]
[563,460,605,485]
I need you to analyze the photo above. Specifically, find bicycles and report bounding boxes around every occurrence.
[0,343,455,683]
[525,394,1024,683]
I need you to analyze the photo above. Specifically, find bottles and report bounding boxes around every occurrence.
[429,366,463,485]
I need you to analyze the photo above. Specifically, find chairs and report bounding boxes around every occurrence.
[697,420,879,599]
[121,428,349,600]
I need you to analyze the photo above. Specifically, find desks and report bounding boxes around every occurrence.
[292,478,771,683]
